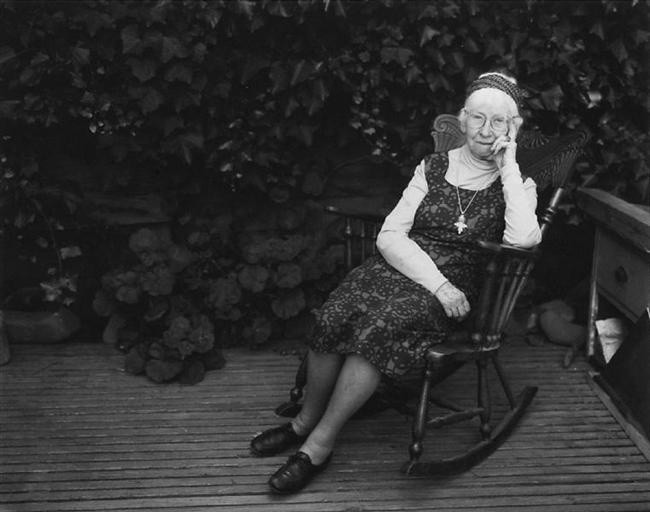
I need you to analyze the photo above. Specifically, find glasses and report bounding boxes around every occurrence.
[463,110,509,132]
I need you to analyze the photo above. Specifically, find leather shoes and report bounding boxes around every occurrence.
[250,422,310,457]
[268,452,332,495]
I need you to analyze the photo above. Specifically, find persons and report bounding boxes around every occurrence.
[250,70,541,499]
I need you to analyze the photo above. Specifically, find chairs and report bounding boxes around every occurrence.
[275,112,592,477]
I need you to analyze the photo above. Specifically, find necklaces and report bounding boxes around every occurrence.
[450,146,496,235]
